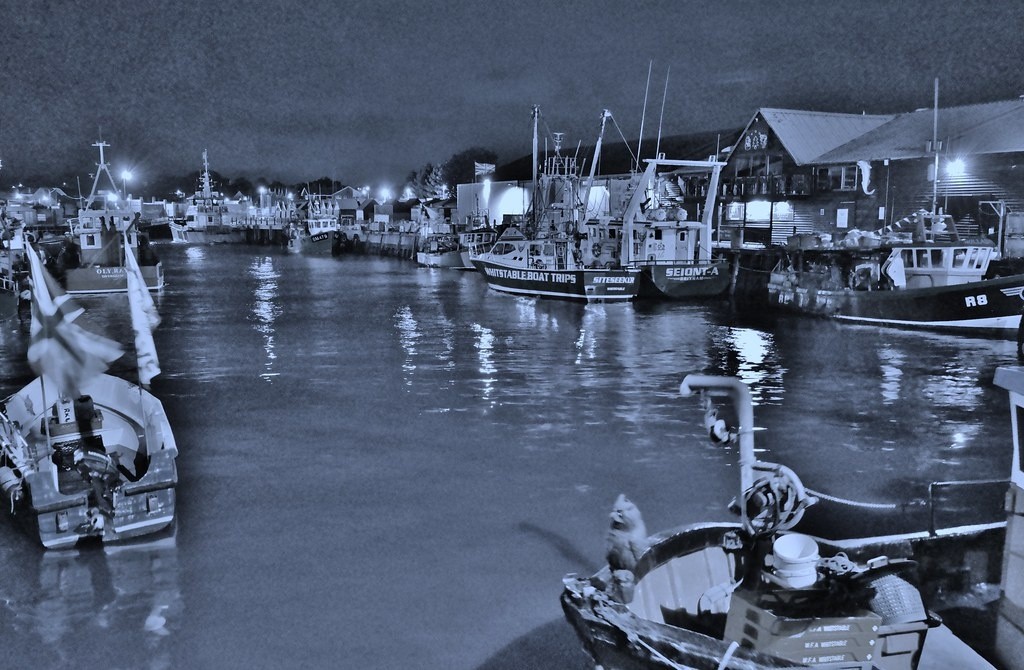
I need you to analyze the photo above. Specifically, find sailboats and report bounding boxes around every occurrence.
[469,103,730,304]
[64,127,164,293]
[166,149,243,244]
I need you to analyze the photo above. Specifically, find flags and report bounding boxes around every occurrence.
[24,237,125,395]
[119,240,162,383]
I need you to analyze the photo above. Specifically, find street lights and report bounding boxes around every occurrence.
[121,171,131,195]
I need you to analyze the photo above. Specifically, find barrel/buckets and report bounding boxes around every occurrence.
[773,535,820,585]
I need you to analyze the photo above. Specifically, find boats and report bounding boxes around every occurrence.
[765,215,1024,339]
[417,228,498,270]
[1,237,179,551]
[289,200,346,255]
[0,228,79,293]
[560,364,1023,670]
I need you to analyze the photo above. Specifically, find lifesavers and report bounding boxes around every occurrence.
[565,220,575,236]
[592,243,601,258]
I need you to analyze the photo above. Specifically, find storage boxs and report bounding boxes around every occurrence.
[41,409,104,437]
[723,592,882,670]
[873,610,943,670]
